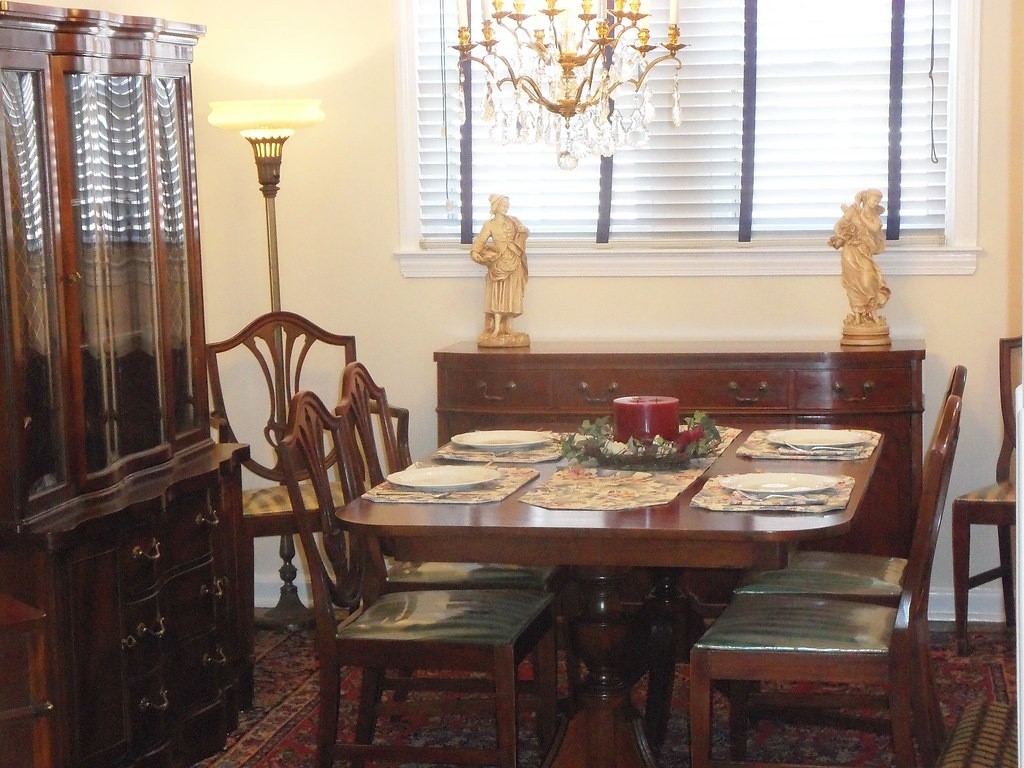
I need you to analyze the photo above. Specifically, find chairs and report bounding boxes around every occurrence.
[952,336,1023,656]
[334,360,579,699]
[690,395,964,768]
[204,312,369,706]
[275,390,560,768]
[732,364,968,726]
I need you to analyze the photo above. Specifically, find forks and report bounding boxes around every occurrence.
[735,489,826,503]
[783,442,856,453]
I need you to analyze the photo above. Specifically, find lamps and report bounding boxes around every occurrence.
[451,1,687,168]
[208,98,325,630]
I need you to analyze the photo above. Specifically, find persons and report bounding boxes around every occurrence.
[828,188,892,326]
[471,193,531,338]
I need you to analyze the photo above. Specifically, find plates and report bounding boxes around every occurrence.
[718,472,838,495]
[386,465,500,491]
[451,430,552,450]
[767,429,872,450]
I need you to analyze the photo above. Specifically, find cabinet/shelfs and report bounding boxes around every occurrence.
[0,1,260,768]
[434,340,927,617]
